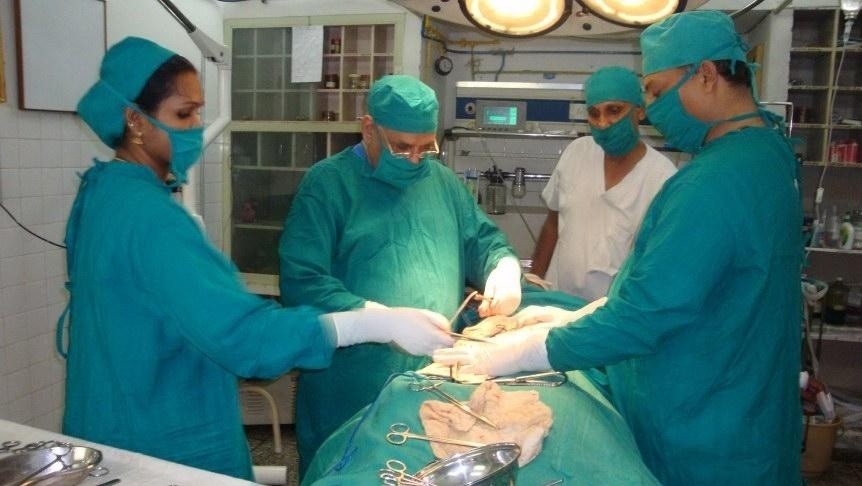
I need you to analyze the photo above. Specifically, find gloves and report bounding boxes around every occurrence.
[477,258,523,318]
[330,300,453,355]
[434,326,553,376]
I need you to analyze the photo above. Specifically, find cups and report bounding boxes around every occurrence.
[348,73,361,88]
[322,110,336,121]
[359,80,369,89]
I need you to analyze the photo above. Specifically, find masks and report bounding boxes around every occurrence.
[589,110,637,158]
[644,91,710,156]
[168,129,203,188]
[362,150,434,191]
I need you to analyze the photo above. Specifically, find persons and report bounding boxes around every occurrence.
[431,12,803,486]
[55,35,454,480]
[276,74,522,486]
[528,64,679,301]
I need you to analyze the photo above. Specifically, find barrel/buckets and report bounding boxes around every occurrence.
[824,277,852,327]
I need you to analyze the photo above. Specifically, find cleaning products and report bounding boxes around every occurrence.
[837,211,855,250]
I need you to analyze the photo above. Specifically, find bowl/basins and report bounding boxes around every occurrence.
[409,443,522,485]
[0,444,104,485]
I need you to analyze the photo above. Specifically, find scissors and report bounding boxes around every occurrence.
[446,331,487,342]
[386,423,488,448]
[409,377,497,428]
[0,440,121,486]
[463,371,566,386]
[380,460,440,486]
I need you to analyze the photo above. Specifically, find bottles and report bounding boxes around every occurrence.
[801,275,847,327]
[795,106,809,123]
[830,141,862,162]
[805,203,862,251]
[485,182,506,215]
[324,73,338,88]
[330,37,340,53]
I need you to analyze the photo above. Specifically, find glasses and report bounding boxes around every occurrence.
[376,125,440,159]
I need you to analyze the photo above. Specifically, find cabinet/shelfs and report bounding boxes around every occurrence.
[224,18,402,305]
[797,240,861,345]
[789,10,861,170]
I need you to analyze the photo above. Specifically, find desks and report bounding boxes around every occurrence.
[0,416,276,486]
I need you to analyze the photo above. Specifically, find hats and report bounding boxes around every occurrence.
[365,76,441,134]
[584,66,645,113]
[80,37,177,147]
[640,11,748,75]
